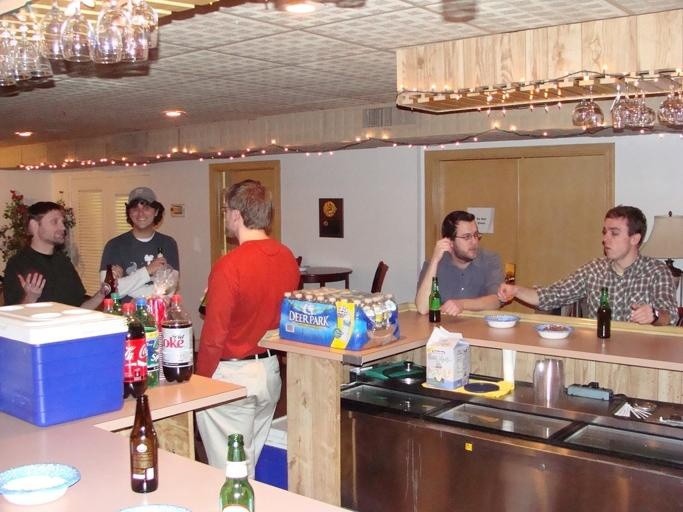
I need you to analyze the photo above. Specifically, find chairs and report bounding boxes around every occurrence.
[369,262,389,292]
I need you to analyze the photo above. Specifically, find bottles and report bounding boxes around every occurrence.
[428,276,441,323]
[103,263,114,292]
[156,247,164,266]
[103,292,193,398]
[128,394,156,492]
[279,287,399,350]
[596,288,610,340]
[529,359,566,405]
[219,434,254,510]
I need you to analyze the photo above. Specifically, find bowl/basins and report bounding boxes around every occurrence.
[298,264,310,272]
[485,314,519,329]
[535,322,572,339]
[0,465,81,503]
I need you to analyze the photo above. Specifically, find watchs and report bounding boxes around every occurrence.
[651,308,659,324]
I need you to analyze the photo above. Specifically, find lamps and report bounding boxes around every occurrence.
[640,211,682,276]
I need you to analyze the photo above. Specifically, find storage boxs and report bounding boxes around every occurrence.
[0,299,128,429]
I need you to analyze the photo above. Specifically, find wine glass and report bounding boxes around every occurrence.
[573,78,681,130]
[0,3,159,86]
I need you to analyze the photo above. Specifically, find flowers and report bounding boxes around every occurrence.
[0,189,75,277]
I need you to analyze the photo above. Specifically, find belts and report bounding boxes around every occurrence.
[220,349,278,362]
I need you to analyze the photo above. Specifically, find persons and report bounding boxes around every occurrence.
[194,179,301,481]
[98,187,179,304]
[3,202,124,309]
[497,204,680,326]
[415,211,504,317]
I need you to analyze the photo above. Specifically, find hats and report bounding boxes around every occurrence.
[128,187,157,203]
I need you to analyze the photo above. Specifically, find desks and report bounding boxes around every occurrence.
[299,265,354,290]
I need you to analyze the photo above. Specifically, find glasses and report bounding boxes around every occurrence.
[447,231,484,241]
